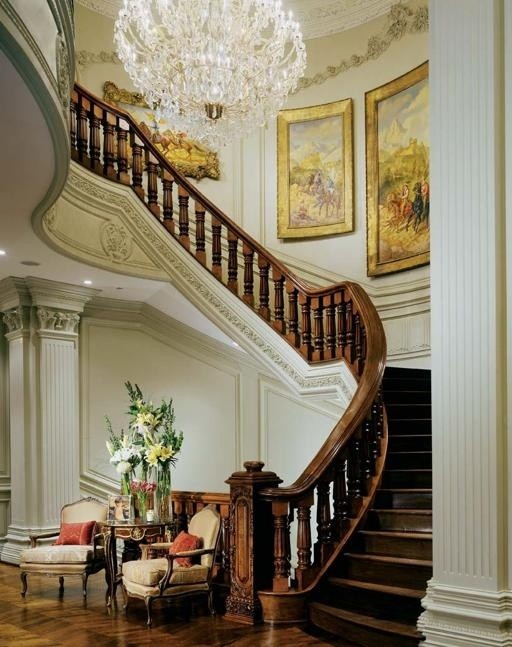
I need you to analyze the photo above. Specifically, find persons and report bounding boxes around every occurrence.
[113,495,129,519]
[109,506,115,520]
[295,168,429,227]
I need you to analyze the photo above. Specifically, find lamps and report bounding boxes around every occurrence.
[109,0,307,138]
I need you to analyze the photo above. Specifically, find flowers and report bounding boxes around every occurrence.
[97,380,191,522]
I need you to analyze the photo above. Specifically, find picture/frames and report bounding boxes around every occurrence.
[276,59,431,278]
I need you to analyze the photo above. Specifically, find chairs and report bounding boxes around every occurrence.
[18,496,223,626]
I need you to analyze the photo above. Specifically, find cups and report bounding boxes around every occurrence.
[146,512,154,522]
